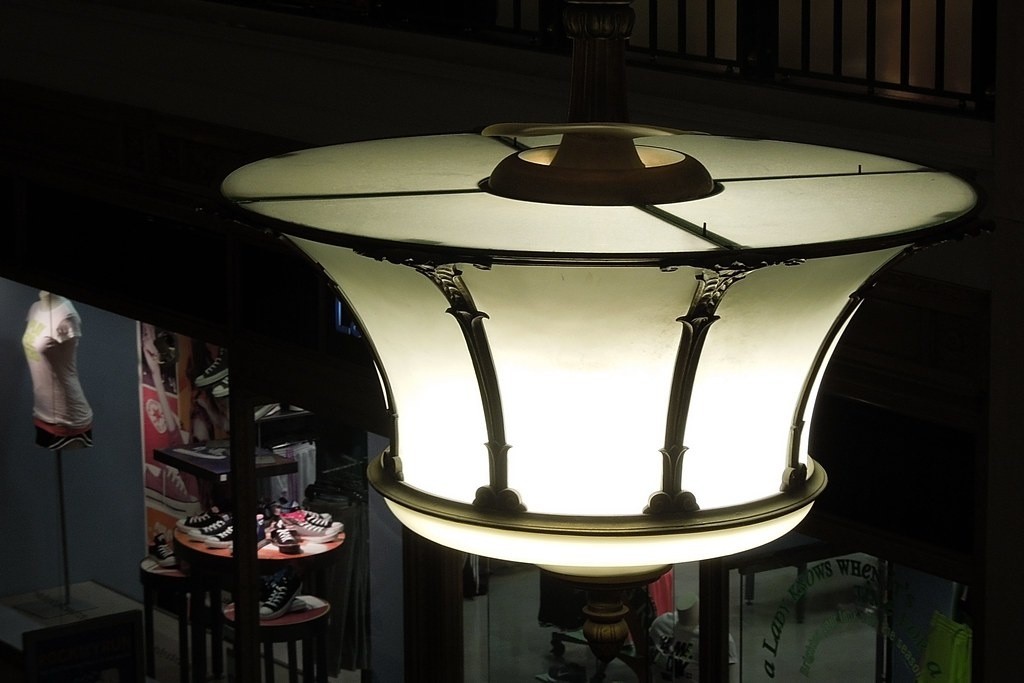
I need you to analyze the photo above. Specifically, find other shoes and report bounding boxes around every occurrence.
[253,522,266,549]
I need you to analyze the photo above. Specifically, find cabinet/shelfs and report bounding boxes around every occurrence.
[153,410,320,481]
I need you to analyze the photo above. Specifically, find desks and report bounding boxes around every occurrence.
[738,541,860,624]
[140,511,346,683]
[544,564,676,683]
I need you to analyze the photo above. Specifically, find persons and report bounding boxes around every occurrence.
[22,292,93,450]
[143,328,228,502]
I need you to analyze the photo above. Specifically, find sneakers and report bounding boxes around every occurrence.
[188,517,233,538]
[175,503,226,529]
[148,533,177,566]
[194,347,229,386]
[271,522,299,548]
[212,376,230,396]
[145,464,200,509]
[205,525,235,548]
[267,498,344,534]
[259,573,303,618]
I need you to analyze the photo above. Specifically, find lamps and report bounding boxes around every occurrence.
[217,0,997,661]
